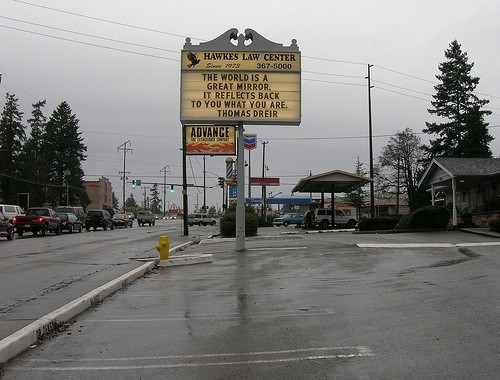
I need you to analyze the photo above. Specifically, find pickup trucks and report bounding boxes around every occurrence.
[13,207,60,237]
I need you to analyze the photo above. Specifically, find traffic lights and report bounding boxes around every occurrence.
[131,180,135,189]
[218,176,224,188]
[170,185,174,192]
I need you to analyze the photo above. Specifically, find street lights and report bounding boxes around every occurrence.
[203,170,225,212]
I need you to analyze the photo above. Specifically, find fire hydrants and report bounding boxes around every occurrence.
[155,235,171,260]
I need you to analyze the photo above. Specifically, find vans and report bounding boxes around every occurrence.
[0,204,26,221]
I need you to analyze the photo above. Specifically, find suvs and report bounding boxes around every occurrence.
[188,213,217,227]
[55,206,87,223]
[300,208,358,228]
[137,211,155,226]
[85,210,114,231]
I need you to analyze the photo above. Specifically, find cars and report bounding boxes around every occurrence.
[153,214,159,219]
[0,212,15,240]
[112,213,134,228]
[272,212,304,227]
[163,214,177,219]
[55,213,83,234]
[126,212,135,220]
